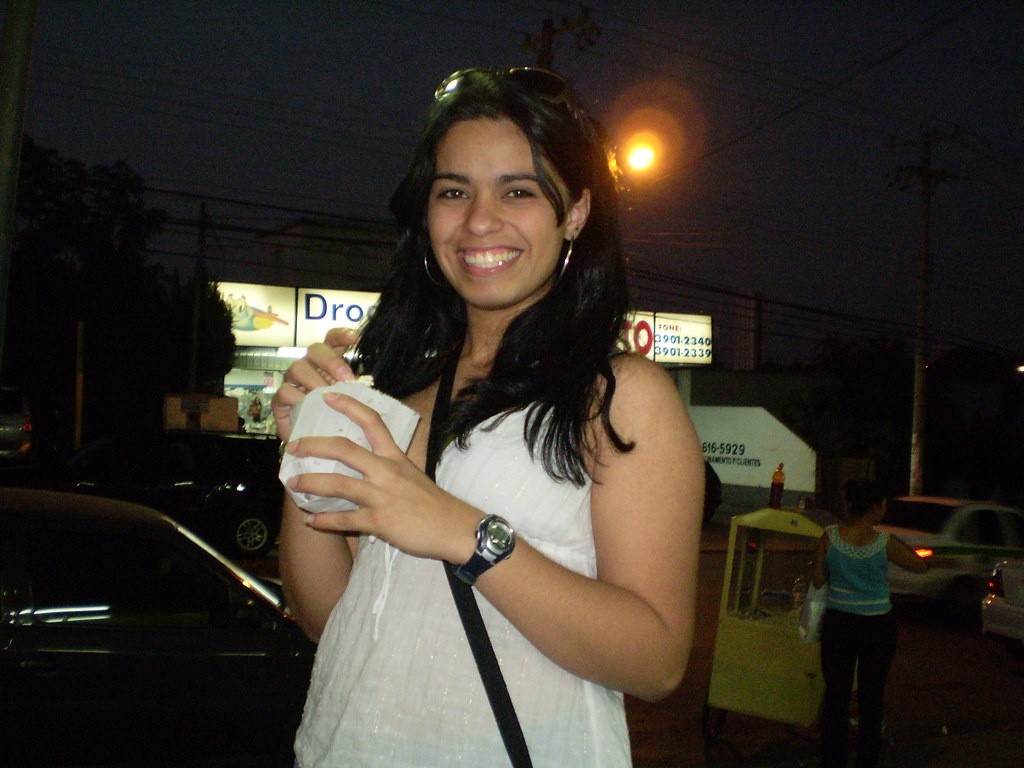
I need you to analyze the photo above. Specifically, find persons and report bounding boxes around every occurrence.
[812,474,930,758]
[272,63,707,768]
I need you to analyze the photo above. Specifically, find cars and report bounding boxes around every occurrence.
[981,559,1024,661]
[0,383,320,768]
[865,496,1024,614]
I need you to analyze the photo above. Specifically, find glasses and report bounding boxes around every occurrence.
[432,63,579,109]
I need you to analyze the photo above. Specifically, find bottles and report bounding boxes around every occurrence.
[768,463,785,509]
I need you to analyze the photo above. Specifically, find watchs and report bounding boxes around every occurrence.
[452,515,517,584]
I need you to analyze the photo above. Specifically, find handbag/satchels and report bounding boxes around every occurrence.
[799,580,831,645]
